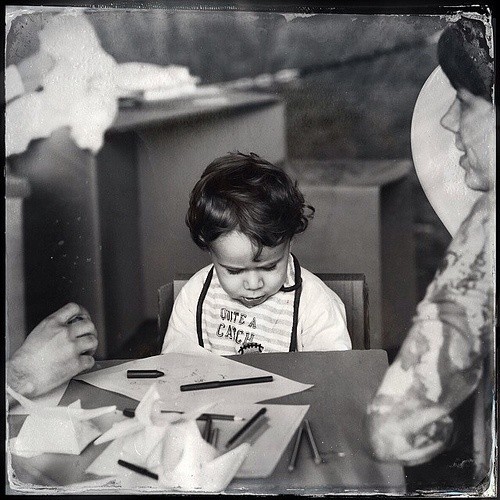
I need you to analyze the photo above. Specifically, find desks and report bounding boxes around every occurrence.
[15,93,289,359]
[277,159,417,350]
[7,349,407,499]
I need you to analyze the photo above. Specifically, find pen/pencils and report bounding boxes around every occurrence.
[118,459,158,480]
[226,408,267,447]
[288,423,303,473]
[180,376,274,391]
[202,420,212,441]
[211,428,219,447]
[305,420,322,466]
[121,409,245,421]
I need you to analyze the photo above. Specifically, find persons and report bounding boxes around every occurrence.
[361,18,496,492]
[161,150,352,355]
